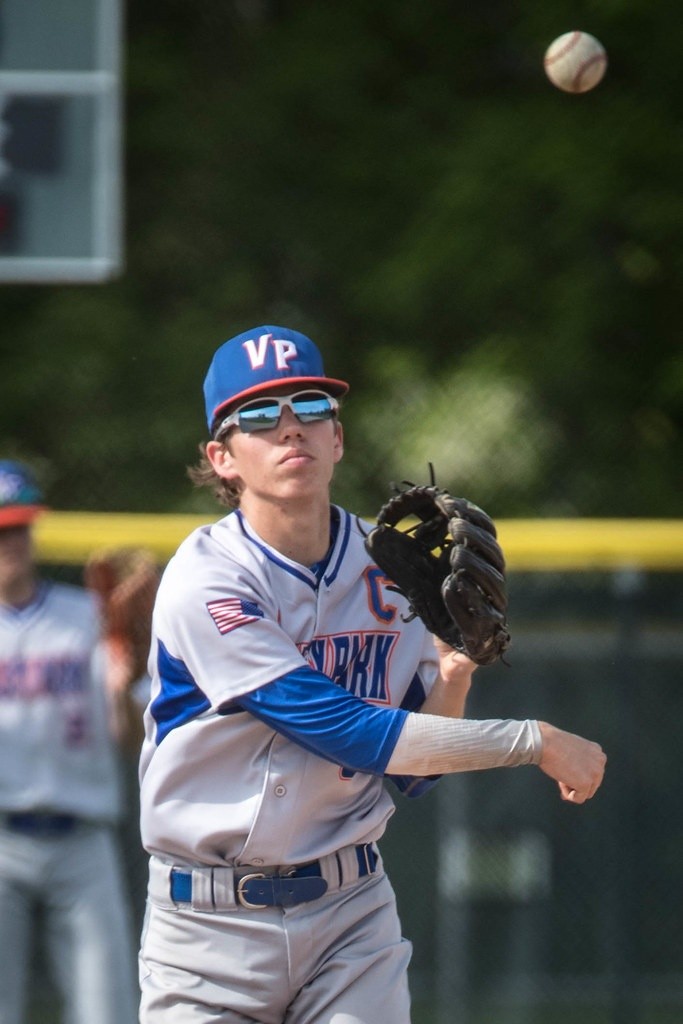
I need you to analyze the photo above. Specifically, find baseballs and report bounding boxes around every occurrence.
[544,28,610,95]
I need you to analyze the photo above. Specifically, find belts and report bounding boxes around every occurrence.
[11,815,76,837]
[171,846,376,906]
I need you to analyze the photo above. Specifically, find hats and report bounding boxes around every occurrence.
[203,326,349,435]
[0,462,40,527]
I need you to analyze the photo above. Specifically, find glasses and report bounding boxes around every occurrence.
[215,389,339,441]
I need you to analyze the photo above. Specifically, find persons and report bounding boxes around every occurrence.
[0,457,160,1024]
[136,322,610,1024]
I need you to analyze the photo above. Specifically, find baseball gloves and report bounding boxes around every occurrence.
[362,482,513,669]
[89,549,160,685]
